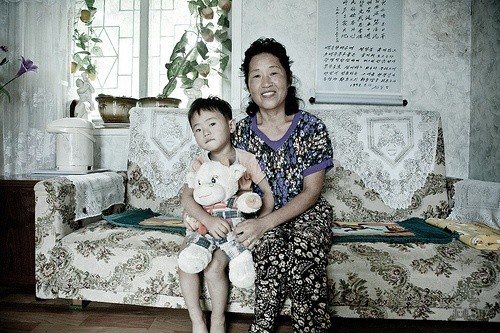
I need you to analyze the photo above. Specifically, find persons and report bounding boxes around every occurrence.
[176,95,274,333]
[74,73,99,119]
[183,78,204,108]
[229,37,334,332]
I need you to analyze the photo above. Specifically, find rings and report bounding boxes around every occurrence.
[247,240,250,244]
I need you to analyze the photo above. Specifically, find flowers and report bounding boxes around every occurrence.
[0,45,38,103]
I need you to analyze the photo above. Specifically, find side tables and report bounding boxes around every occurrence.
[0,174,85,301]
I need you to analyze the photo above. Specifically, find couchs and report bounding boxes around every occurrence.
[34,107,500,322]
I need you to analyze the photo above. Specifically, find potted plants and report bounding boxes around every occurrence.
[71,0,138,122]
[138,0,232,107]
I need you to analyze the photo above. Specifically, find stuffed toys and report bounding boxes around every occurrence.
[178,161,262,288]
